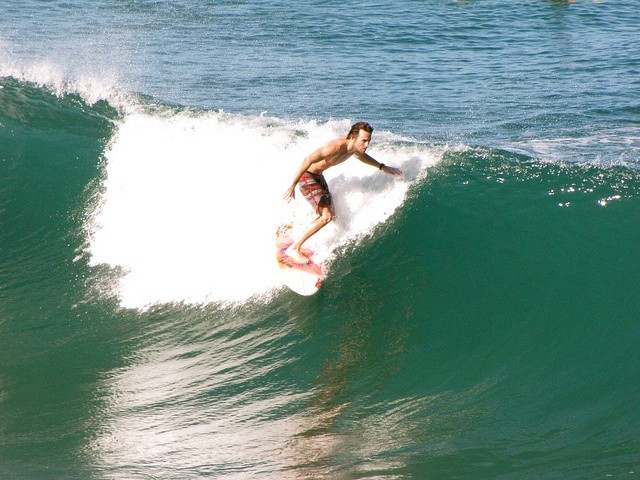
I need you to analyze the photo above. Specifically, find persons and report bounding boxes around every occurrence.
[284,122,402,265]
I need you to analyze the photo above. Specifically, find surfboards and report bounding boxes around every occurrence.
[276,223,325,296]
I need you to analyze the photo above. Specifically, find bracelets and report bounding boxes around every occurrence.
[379,163,385,170]
